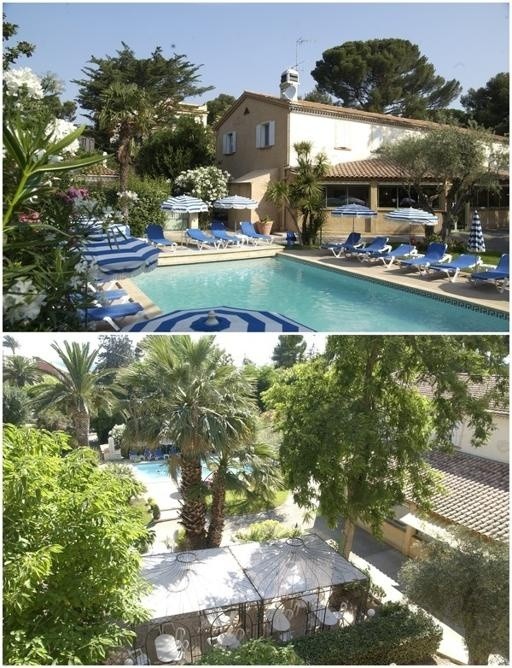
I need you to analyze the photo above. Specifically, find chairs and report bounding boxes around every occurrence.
[280,230,296,246]
[323,232,508,294]
[148,221,272,255]
[67,283,150,330]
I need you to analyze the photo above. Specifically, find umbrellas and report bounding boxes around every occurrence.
[162,195,211,232]
[67,229,164,285]
[326,199,377,233]
[467,210,488,274]
[214,193,258,236]
[119,302,317,332]
[382,206,437,258]
